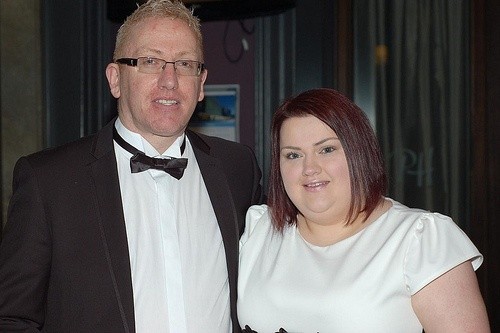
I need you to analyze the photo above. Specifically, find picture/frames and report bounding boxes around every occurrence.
[188,84,240,143]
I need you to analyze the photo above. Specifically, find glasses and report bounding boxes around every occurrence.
[114,57,206,76]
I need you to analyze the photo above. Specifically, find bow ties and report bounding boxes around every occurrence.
[113,123,189,181]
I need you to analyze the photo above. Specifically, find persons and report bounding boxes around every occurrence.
[237,88,490,333]
[0,0,268,333]
